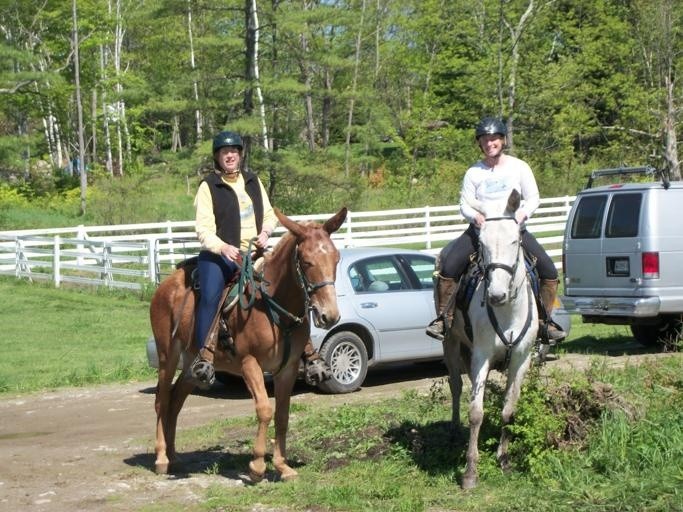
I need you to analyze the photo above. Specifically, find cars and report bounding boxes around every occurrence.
[144,246,571,395]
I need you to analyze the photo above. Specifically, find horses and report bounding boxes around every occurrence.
[148,205,348,489]
[432,185,541,493]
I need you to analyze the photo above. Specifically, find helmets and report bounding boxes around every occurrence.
[474,117,506,138]
[213,131,244,151]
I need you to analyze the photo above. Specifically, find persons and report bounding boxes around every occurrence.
[425,117,567,341]
[192,132,328,389]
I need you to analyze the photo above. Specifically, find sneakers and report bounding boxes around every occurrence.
[303,339,319,363]
[194,361,215,387]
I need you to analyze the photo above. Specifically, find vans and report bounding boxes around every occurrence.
[560,163,683,347]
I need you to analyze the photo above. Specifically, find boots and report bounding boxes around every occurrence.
[425,271,459,333]
[537,277,567,340]
